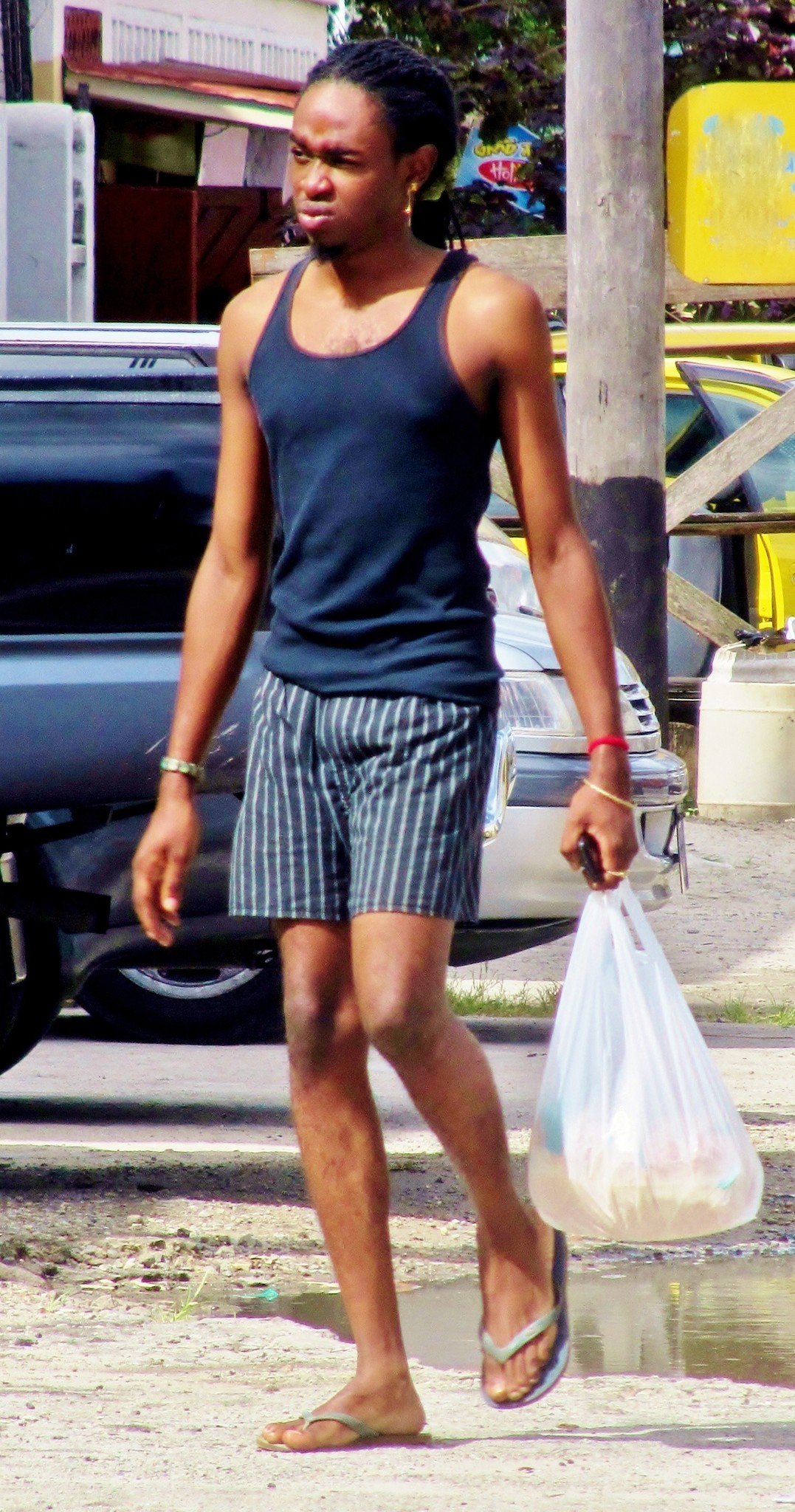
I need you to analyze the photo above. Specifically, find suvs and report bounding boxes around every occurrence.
[0,318,688,1078]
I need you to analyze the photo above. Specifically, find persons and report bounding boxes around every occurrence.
[122,44,646,1458]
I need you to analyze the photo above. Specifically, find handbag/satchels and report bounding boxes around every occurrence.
[525,869,766,1241]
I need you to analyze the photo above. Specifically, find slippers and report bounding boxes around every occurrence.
[255,1409,433,1452]
[478,1227,573,1407]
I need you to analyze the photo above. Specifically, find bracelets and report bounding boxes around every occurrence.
[158,754,207,783]
[586,735,629,755]
[578,772,640,814]
